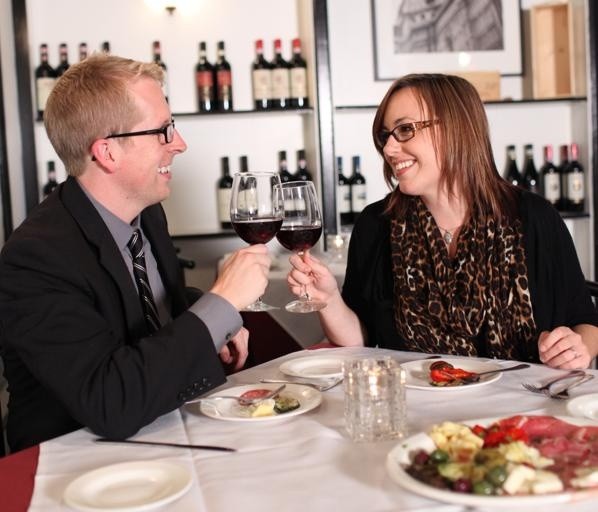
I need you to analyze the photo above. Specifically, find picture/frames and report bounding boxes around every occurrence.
[370,0,524,82]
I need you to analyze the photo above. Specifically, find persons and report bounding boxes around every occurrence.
[0,49,271,453]
[289,73,598,370]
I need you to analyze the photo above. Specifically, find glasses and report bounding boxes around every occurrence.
[381,119,442,143]
[91,117,177,161]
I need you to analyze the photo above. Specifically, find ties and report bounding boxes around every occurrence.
[126,228,162,335]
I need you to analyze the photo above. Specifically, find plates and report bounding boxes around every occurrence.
[61,355,598,512]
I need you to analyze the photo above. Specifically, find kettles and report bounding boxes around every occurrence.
[195,42,214,111]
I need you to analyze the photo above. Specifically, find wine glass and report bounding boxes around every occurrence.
[273,181,325,313]
[229,172,285,312]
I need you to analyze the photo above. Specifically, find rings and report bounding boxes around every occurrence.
[570,348,577,358]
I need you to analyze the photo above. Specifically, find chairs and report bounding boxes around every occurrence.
[584,279,598,305]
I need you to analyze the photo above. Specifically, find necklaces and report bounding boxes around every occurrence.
[438,225,459,243]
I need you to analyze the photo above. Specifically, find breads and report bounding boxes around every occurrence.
[246,397,276,417]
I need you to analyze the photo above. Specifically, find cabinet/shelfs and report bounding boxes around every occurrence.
[12,1,338,252]
[312,0,598,281]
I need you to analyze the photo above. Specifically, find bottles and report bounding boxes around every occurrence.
[150,39,169,107]
[34,41,111,119]
[213,41,233,112]
[42,161,61,200]
[504,144,585,213]
[250,39,310,111]
[338,156,367,225]
[215,150,314,230]
